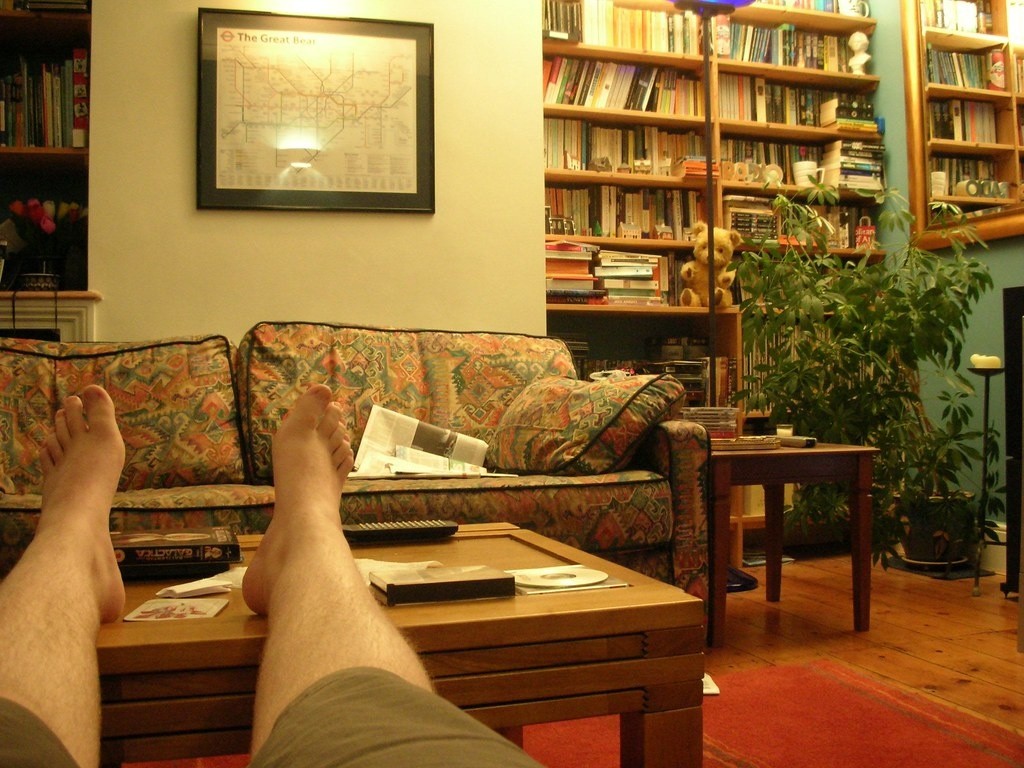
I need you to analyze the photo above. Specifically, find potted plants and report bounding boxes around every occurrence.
[733,173,1010,568]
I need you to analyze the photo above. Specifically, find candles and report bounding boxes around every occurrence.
[970,352,1000,367]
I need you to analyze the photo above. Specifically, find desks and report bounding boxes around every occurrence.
[95,521,706,767]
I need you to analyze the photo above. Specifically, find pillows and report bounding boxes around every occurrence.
[486,373,685,477]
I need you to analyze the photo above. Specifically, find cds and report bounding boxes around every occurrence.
[512,566,608,587]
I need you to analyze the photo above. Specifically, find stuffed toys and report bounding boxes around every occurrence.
[681,220,740,306]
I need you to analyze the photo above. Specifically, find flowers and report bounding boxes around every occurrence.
[8,197,88,273]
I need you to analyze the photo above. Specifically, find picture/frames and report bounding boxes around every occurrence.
[195,8,435,212]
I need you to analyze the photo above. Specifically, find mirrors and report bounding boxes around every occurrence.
[898,0,1023,250]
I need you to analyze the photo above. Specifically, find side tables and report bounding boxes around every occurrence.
[707,439,881,648]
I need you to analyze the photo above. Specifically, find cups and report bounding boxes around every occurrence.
[776,423,793,437]
[792,160,825,187]
[931,172,946,197]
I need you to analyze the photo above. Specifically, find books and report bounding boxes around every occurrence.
[541,0,887,451]
[0,49,88,149]
[919,0,1024,218]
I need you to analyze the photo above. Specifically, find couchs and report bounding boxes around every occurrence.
[0,322,709,641]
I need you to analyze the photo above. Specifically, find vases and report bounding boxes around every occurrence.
[21,273,63,292]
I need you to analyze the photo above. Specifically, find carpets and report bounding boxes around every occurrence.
[115,657,1023,768]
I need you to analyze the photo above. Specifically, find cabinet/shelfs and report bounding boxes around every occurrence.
[0,1,91,172]
[542,0,888,563]
[917,0,1023,222]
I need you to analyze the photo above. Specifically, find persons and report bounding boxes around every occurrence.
[0,383,542,768]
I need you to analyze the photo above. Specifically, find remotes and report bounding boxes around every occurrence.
[341,520,459,542]
[778,436,818,448]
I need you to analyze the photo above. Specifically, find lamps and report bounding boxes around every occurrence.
[672,1,755,406]
